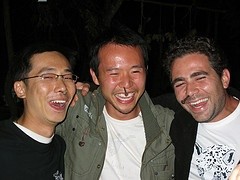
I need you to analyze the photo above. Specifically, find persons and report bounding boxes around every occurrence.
[55,32,176,180]
[0,43,76,180]
[70,34,240,180]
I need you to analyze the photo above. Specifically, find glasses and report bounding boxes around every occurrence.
[21,72,79,83]
